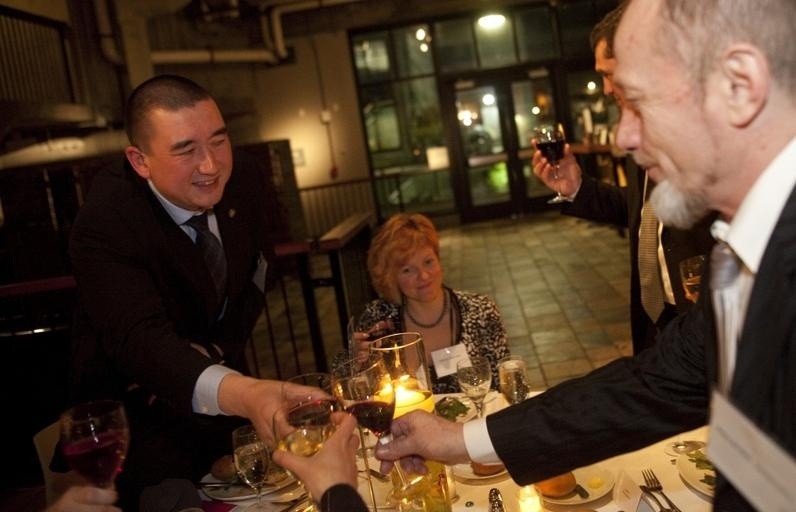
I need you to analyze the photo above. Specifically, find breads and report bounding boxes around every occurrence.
[211,455,238,481]
[469,461,505,477]
[534,471,576,499]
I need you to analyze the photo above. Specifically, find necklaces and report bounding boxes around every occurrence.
[404,289,450,329]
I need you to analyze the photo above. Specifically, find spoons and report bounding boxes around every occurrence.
[639,485,670,511]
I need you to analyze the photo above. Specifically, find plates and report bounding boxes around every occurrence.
[677,448,717,499]
[450,464,508,479]
[201,473,295,502]
[535,464,615,506]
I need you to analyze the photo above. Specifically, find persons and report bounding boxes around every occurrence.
[332,215,512,401]
[530,4,719,358]
[376,0,796,511]
[1,484,122,512]
[64,73,332,510]
[271,411,372,511]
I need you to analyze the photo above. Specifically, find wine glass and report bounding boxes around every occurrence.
[533,122,573,205]
[333,356,410,491]
[62,398,132,488]
[266,401,323,512]
[498,355,529,405]
[231,423,270,512]
[282,370,343,428]
[457,356,492,417]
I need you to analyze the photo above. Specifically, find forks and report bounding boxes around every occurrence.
[640,468,681,512]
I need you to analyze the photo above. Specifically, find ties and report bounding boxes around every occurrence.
[708,242,743,389]
[636,199,665,325]
[188,212,228,293]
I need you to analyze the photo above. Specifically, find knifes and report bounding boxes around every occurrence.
[202,478,275,489]
